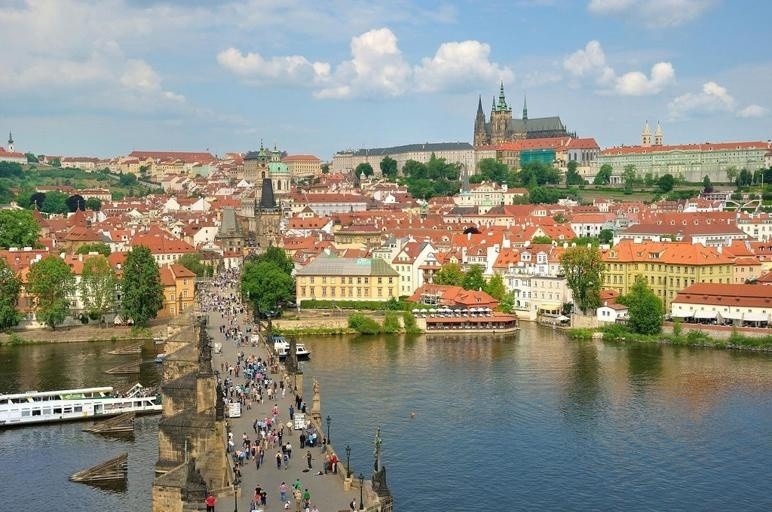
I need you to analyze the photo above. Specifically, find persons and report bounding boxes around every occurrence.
[198,266,356,512]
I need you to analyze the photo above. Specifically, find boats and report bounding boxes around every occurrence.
[271,336,311,358]
[0,381,163,429]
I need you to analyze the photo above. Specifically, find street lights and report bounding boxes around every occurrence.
[345,444,352,478]
[231,477,241,512]
[325,414,331,445]
[358,472,365,511]
